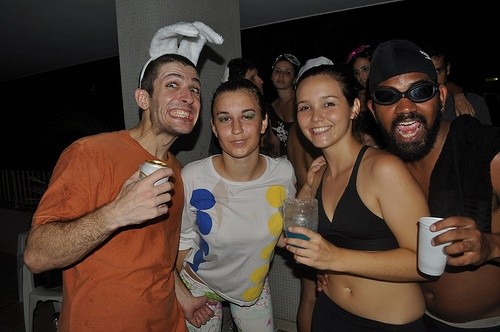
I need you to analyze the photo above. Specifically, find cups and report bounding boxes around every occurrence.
[418,217,457,276]
[282,198,319,241]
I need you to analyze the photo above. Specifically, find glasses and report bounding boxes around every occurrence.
[436,66,447,74]
[371,80,439,105]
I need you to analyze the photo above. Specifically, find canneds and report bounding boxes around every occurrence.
[138,159,169,186]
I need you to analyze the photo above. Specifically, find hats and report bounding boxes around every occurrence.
[369,40,438,94]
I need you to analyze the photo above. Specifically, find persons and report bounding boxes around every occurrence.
[207,37,492,332]
[175,79,297,332]
[316,41,500,332]
[284,65,436,331]
[23,21,225,332]
